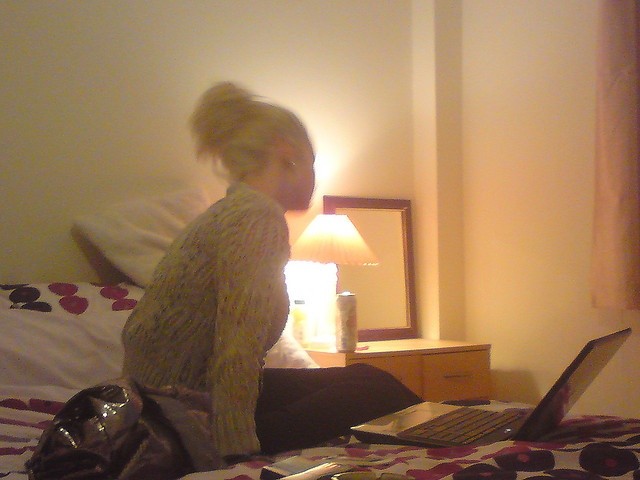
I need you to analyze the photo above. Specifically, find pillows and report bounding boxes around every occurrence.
[0,310,129,385]
[73,191,320,368]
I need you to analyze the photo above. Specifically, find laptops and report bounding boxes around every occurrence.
[348,325,634,449]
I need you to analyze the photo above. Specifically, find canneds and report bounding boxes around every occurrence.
[336,294,357,353]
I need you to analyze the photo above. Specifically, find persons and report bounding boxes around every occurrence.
[122,83,424,470]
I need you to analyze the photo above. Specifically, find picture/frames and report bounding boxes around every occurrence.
[323,194,418,342]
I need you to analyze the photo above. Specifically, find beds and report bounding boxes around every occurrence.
[1,279,640,480]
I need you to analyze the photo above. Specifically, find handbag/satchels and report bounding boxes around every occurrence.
[24,375,188,479]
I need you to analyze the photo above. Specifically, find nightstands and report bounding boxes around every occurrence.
[297,337,494,404]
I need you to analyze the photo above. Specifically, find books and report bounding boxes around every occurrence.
[258,457,330,479]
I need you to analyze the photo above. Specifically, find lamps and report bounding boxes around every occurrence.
[288,213,380,293]
